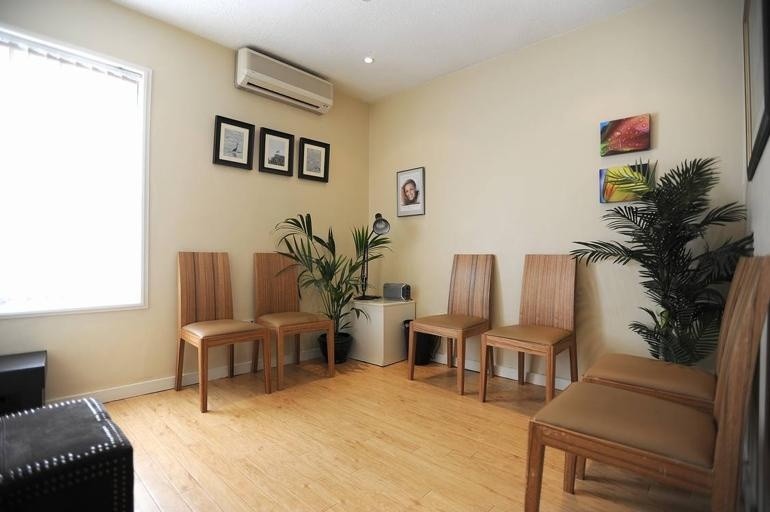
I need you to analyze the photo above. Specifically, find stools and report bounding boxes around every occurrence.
[0,349,48,417]
[1,395,134,512]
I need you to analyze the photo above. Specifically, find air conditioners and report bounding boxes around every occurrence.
[234,45,334,115]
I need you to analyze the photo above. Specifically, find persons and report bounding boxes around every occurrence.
[401,177,419,204]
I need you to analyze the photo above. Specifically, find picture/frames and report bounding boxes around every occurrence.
[298,136,331,182]
[396,167,426,216]
[742,0,770,182]
[259,126,295,178]
[213,115,258,171]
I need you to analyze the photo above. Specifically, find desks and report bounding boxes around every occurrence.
[344,296,417,368]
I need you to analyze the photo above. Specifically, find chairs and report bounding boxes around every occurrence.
[478,251,578,403]
[408,253,496,397]
[573,254,768,483]
[521,253,768,512]
[173,250,276,415]
[250,252,337,390]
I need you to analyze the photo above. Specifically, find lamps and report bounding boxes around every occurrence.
[353,213,394,300]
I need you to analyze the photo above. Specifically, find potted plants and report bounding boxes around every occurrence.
[273,213,393,365]
